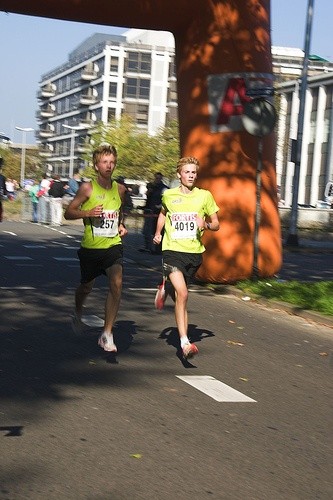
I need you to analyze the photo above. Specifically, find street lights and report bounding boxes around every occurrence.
[15,127,35,188]
[62,124,87,178]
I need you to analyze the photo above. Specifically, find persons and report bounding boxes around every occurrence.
[155,157,220,361]
[137,172,170,253]
[63,145,128,352]
[23,172,79,226]
[116,176,134,224]
[0,157,21,222]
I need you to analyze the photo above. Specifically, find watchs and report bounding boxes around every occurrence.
[205,220,210,229]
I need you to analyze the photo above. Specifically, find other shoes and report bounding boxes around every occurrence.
[71,313,82,334]
[182,343,198,360]
[98,333,118,353]
[154,282,167,310]
